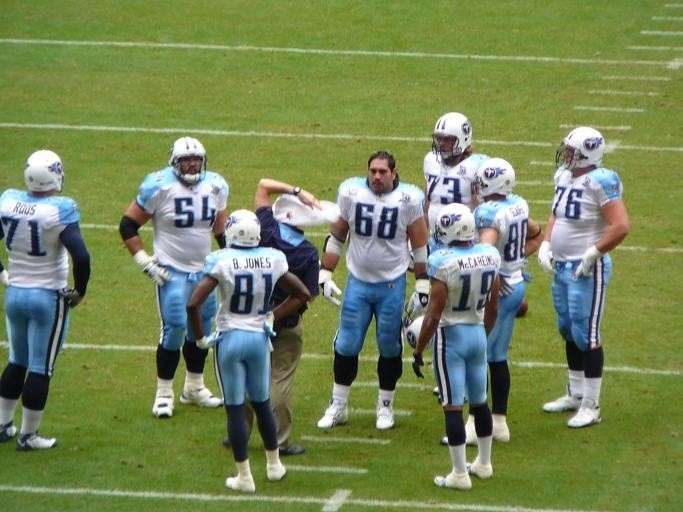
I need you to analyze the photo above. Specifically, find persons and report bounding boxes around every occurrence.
[439,157,545,445]
[222,179,321,457]
[187,208,312,493]
[423,111,492,253]
[537,125,630,428]
[1,149,88,451]
[411,202,500,490]
[317,151,430,431]
[117,136,228,419]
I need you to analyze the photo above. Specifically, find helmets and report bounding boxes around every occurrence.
[435,203,475,245]
[24,150,64,193]
[224,209,261,248]
[433,112,473,160]
[403,314,432,350]
[476,158,515,199]
[170,137,206,184]
[555,127,605,171]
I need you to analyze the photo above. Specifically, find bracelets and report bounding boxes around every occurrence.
[132,250,150,267]
[214,232,226,250]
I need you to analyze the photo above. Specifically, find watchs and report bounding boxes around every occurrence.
[293,186,300,194]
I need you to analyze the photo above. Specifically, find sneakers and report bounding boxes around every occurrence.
[567,405,602,427]
[153,390,174,417]
[16,432,57,451]
[223,437,232,447]
[543,393,584,412]
[0,421,17,442]
[226,477,255,493]
[279,446,305,454]
[318,399,348,428]
[376,404,395,429]
[267,465,286,481]
[433,386,510,489]
[180,385,223,408]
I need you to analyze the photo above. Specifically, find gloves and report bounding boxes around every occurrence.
[264,311,277,337]
[132,250,173,286]
[412,352,425,379]
[62,288,83,309]
[196,331,224,349]
[537,241,559,275]
[522,258,532,282]
[499,273,514,297]
[576,245,601,279]
[407,278,430,313]
[318,269,342,307]
